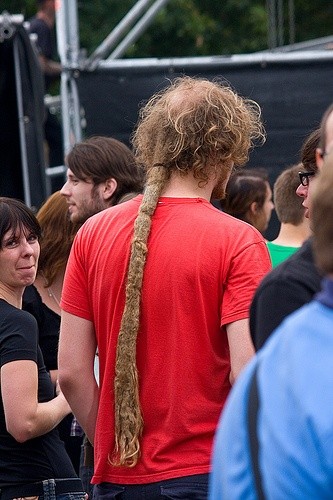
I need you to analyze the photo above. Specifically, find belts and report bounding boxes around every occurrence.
[0,480,84,498]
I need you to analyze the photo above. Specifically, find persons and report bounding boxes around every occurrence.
[0,76,333,500]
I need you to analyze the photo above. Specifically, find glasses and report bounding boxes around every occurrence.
[298,170,314,186]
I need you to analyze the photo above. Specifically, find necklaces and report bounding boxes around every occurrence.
[41,271,60,306]
